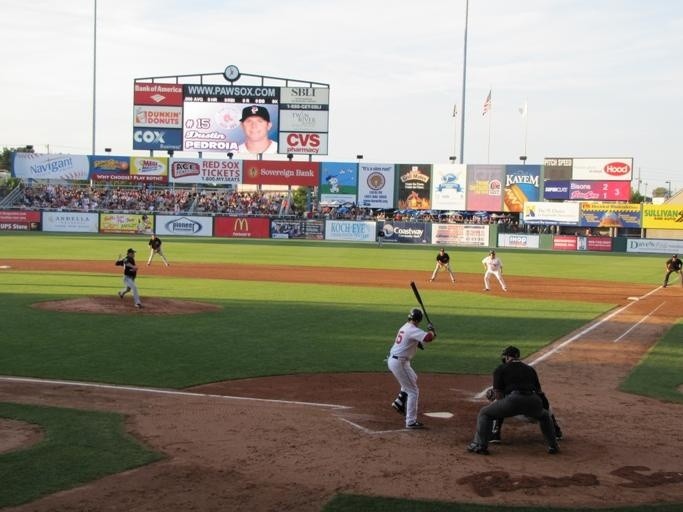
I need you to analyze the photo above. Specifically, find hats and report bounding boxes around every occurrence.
[237,105,271,123]
[127,248,136,254]
[502,345,522,360]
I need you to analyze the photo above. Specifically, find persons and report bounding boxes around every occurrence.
[388,307,438,428]
[481,249,508,292]
[662,254,683,288]
[16,185,287,220]
[466,345,560,454]
[288,202,385,222]
[114,248,144,309]
[485,351,563,445]
[428,247,456,284]
[146,234,169,267]
[230,106,278,153]
[392,209,554,233]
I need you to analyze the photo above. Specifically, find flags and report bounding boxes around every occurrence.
[517,97,526,119]
[452,104,458,117]
[482,92,489,116]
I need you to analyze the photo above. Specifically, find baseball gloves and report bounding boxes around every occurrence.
[486,389,496,401]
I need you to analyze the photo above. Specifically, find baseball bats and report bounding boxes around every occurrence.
[410,282,432,324]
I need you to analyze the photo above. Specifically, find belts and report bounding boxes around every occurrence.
[391,355,409,361]
[511,389,535,395]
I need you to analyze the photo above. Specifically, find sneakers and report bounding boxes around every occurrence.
[554,430,564,440]
[465,440,488,454]
[489,432,502,443]
[545,441,560,454]
[405,420,424,429]
[391,397,407,417]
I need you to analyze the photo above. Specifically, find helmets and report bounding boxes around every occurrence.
[407,308,423,322]
